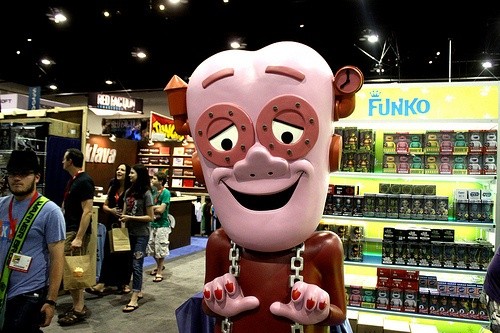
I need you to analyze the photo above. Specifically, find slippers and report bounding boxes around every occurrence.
[150,266,165,275]
[153,277,163,282]
[85,287,104,297]
[129,295,143,302]
[122,285,131,293]
[122,304,138,312]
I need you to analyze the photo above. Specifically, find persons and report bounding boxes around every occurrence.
[57,148,95,327]
[189,195,222,237]
[164,41,363,333]
[114,164,154,315]
[85,162,133,296]
[0,149,66,333]
[144,171,172,282]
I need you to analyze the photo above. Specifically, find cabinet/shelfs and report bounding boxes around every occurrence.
[139,139,222,239]
[318,77,500,333]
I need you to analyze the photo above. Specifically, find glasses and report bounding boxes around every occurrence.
[5,171,37,177]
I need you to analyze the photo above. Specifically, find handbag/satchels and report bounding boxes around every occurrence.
[63,247,96,290]
[109,223,132,253]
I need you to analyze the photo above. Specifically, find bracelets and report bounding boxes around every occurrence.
[45,299,56,306]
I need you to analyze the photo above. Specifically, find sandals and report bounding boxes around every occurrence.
[58,305,87,326]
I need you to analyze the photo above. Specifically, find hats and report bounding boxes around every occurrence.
[5,150,38,170]
